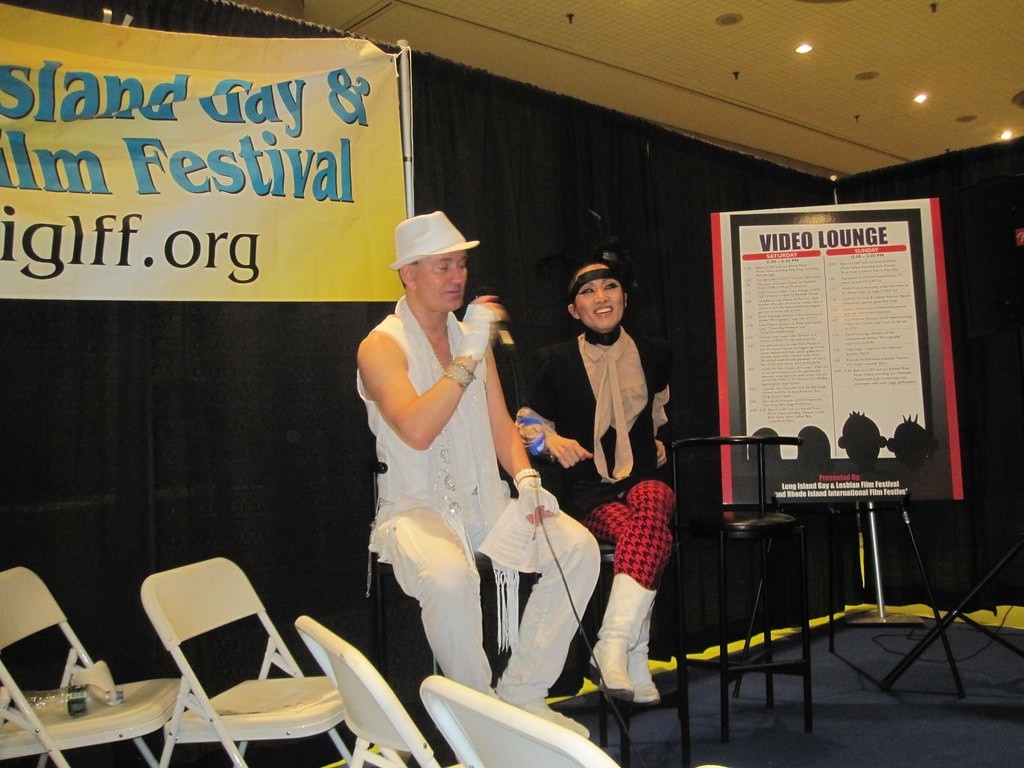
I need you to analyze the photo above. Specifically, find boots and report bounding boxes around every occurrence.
[589,574,660,706]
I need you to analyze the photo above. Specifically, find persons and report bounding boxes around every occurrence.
[356,211,601,740]
[513,259,678,707]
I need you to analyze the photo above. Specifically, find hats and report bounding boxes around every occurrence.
[391,210,481,270]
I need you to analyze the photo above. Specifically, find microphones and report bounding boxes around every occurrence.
[497,323,515,349]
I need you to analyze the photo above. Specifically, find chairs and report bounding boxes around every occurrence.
[419,674,729,768]
[294,615,466,768]
[0,566,181,768]
[351,435,814,768]
[141,557,352,768]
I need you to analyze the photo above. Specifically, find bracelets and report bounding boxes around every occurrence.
[443,359,476,388]
[513,468,540,487]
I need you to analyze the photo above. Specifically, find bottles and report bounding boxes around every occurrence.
[14,683,126,722]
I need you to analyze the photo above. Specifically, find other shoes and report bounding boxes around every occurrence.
[485,686,590,739]
